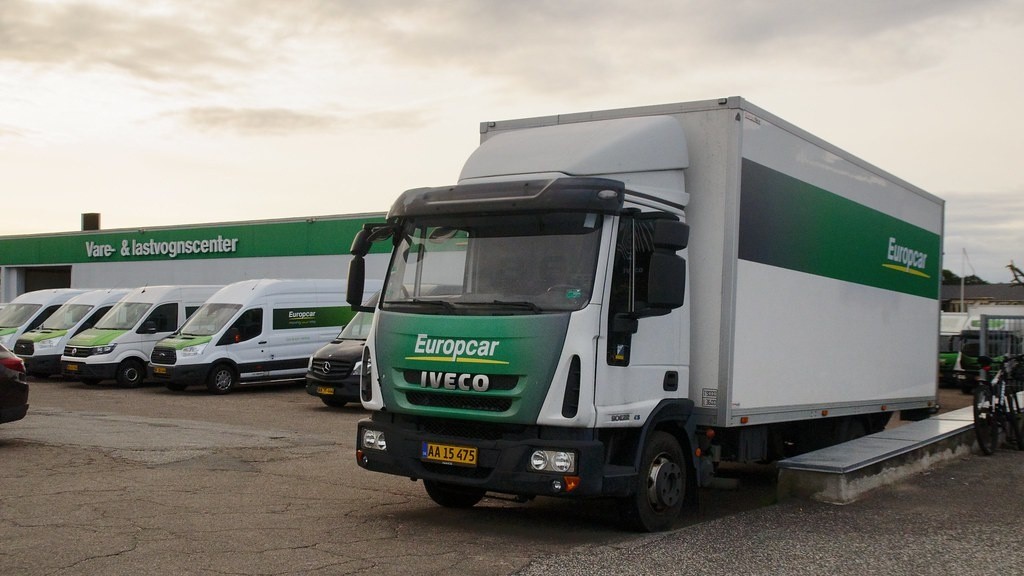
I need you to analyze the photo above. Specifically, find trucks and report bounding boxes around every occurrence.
[346,95,944,534]
[938,306,1024,394]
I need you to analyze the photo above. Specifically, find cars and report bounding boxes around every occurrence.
[0,343,30,424]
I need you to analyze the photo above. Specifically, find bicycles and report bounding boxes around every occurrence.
[973,354,1024,456]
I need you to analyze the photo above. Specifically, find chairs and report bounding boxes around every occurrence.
[475,251,525,295]
[525,254,584,297]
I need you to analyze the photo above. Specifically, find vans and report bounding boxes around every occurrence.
[142,279,387,395]
[0,286,229,389]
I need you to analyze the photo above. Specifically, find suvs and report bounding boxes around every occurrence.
[305,284,464,402]
